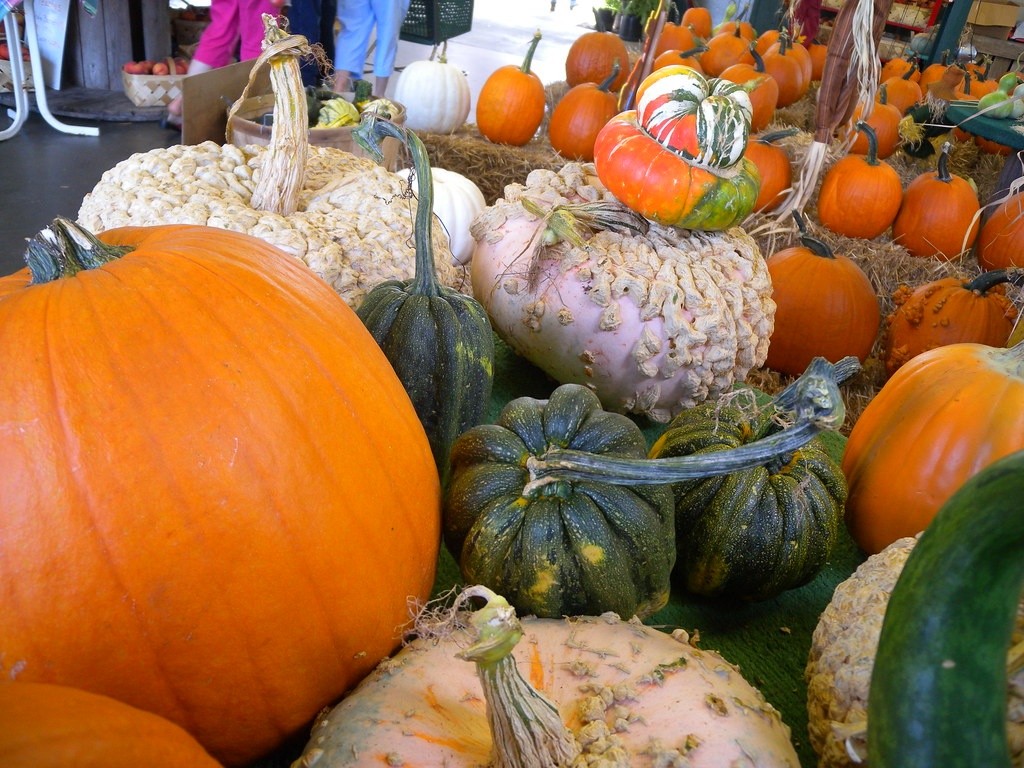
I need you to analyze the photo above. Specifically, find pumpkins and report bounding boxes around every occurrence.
[0,0,1024,768]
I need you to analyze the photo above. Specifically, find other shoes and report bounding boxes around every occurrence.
[161,105,183,131]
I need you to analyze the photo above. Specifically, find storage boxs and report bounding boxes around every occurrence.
[122,56,188,107]
[0,37,36,93]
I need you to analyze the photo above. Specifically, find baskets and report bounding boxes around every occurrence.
[121,56,187,107]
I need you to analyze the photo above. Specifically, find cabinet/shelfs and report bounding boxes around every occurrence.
[813,0,943,67]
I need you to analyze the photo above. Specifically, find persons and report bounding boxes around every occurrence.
[331,0,409,102]
[159,0,281,134]
[286,0,338,87]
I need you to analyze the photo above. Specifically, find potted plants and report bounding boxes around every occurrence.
[618,1,657,42]
[592,0,621,32]
